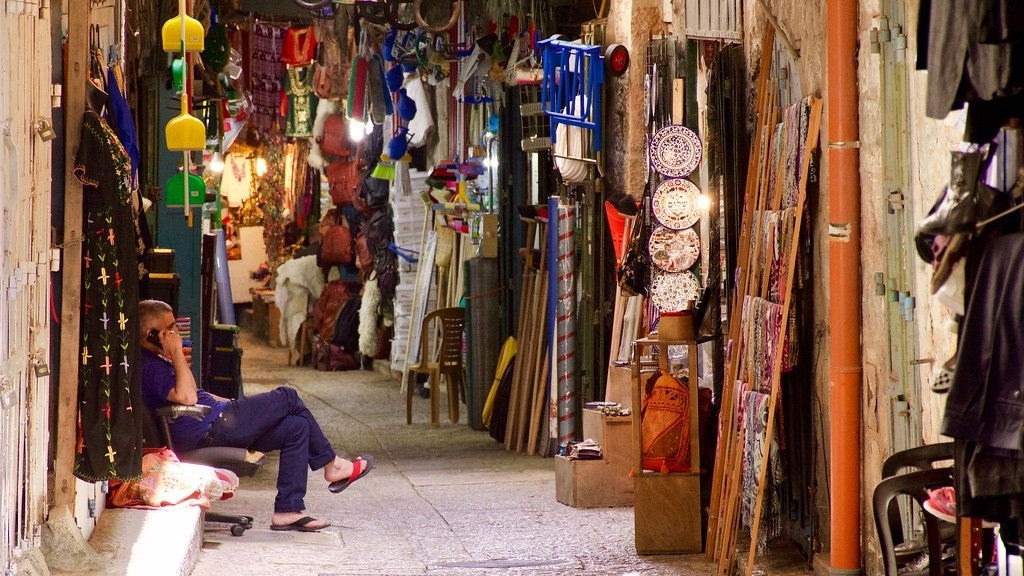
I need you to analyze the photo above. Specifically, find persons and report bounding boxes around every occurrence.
[137,299,375,531]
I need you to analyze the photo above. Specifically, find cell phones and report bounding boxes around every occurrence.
[146,330,163,349]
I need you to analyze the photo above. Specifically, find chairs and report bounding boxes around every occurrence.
[873,442,958,576]
[406,307,466,429]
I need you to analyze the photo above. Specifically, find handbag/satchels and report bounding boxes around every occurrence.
[313,64,350,99]
[322,115,349,157]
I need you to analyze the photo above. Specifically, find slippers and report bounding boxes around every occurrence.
[270,516,331,531]
[328,454,374,493]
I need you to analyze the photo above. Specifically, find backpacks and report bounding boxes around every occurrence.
[312,157,395,355]
